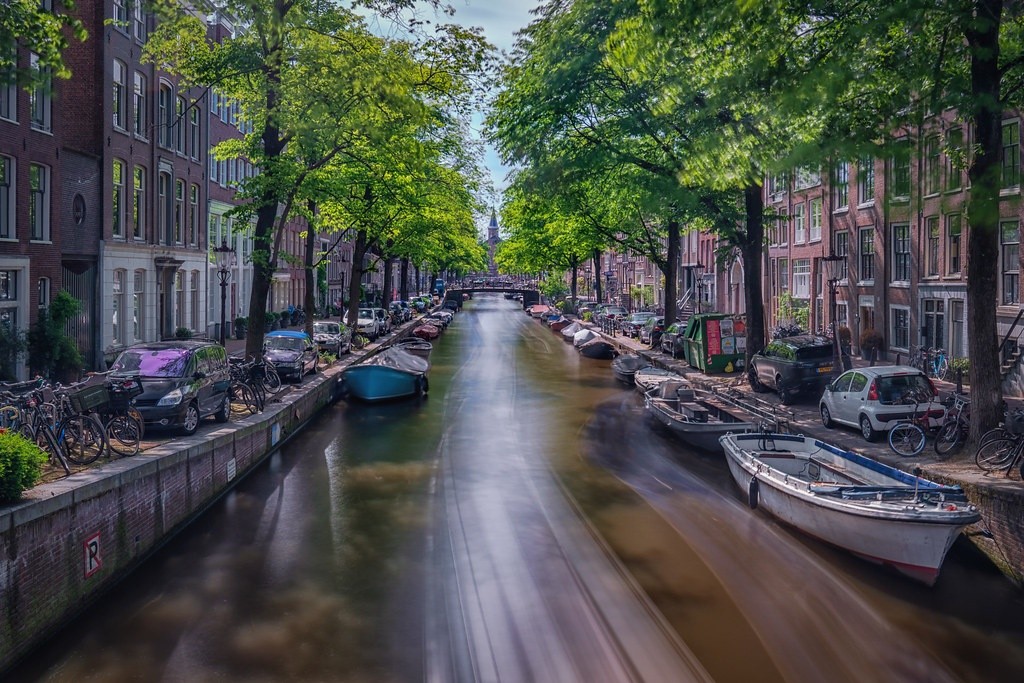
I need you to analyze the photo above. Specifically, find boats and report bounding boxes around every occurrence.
[717,423,982,590]
[525,302,615,359]
[340,337,433,407]
[610,353,777,456]
[411,300,458,340]
[503,292,523,303]
[463,293,468,301]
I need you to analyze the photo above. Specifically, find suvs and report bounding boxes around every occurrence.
[107,338,233,437]
[748,333,853,405]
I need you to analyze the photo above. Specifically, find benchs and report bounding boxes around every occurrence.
[750,450,875,486]
[705,399,754,422]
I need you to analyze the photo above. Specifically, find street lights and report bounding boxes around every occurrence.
[691,260,707,313]
[337,255,347,325]
[213,237,233,348]
[420,266,426,294]
[820,251,845,386]
[584,269,592,302]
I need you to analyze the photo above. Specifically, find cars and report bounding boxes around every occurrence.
[574,299,687,359]
[819,366,948,442]
[300,320,351,359]
[264,331,319,382]
[343,289,439,342]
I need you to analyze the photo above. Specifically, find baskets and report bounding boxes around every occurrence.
[69,384,109,413]
[1004,410,1024,435]
[106,377,144,404]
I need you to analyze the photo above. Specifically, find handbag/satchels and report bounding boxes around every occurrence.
[251,366,265,379]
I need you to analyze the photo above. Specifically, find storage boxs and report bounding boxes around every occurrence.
[69,385,110,412]
[109,377,144,402]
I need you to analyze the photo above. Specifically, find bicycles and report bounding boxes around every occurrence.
[0,369,144,475]
[341,322,363,348]
[909,343,948,381]
[222,344,282,414]
[888,387,1024,479]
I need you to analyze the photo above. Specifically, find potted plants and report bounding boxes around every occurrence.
[273,312,282,330]
[838,326,851,354]
[280,311,291,328]
[264,313,275,333]
[859,329,883,361]
[235,318,247,340]
[291,310,301,327]
